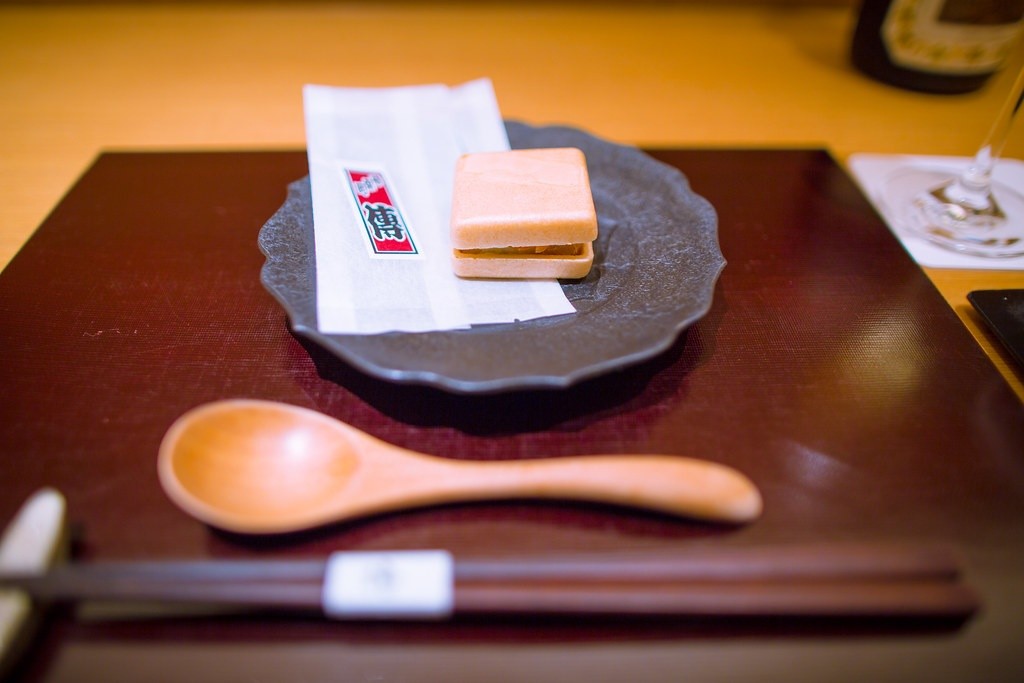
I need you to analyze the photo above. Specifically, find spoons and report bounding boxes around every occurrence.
[156,397,763,533]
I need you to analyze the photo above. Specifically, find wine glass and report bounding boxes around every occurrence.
[876,65,1022,261]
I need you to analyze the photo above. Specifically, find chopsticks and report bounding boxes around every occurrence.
[30,548,981,634]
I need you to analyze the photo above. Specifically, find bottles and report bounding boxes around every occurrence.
[849,0,1024,93]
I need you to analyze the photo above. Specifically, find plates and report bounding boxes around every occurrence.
[258,114,727,400]
